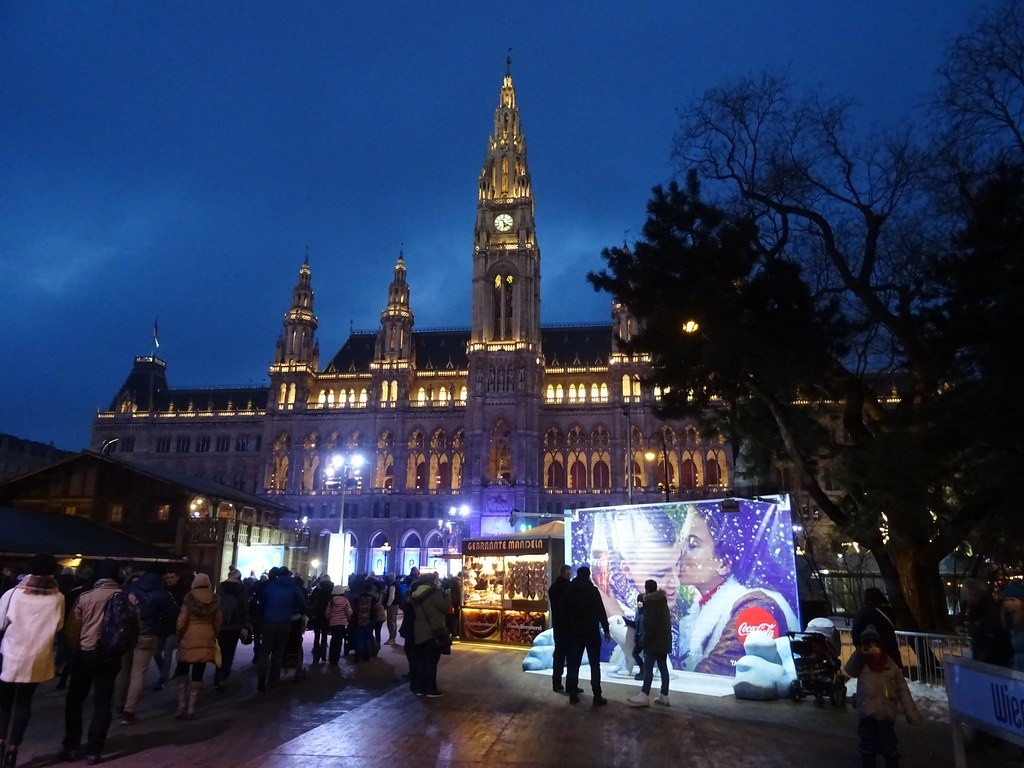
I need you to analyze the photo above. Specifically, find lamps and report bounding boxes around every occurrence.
[505,509,577,528]
[721,492,785,512]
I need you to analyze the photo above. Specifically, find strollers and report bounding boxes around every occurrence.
[787,617,852,708]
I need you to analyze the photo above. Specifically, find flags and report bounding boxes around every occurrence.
[154,320,159,349]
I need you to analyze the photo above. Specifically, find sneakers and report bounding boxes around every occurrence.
[627,691,650,708]
[654,693,670,708]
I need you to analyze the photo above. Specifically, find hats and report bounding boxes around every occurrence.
[93,559,123,585]
[332,586,346,594]
[1003,582,1024,603]
[855,624,881,644]
[864,587,888,607]
[61,567,73,575]
[28,552,57,575]
[229,565,240,580]
[190,573,211,589]
[418,573,437,585]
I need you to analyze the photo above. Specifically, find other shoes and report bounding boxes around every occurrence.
[417,690,425,697]
[569,696,579,704]
[426,690,444,698]
[592,698,608,706]
[636,671,653,680]
[54,638,411,764]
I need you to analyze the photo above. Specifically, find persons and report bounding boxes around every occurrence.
[174,566,251,720]
[57,559,141,765]
[548,565,584,693]
[844,587,922,768]
[628,580,672,706]
[306,566,398,664]
[622,594,646,680]
[569,567,610,705]
[399,566,462,698]
[959,579,1024,673]
[115,564,185,725]
[0,553,65,768]
[588,500,799,677]
[243,566,307,692]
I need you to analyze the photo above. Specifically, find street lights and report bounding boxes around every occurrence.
[645,430,669,502]
[323,453,366,585]
[447,504,473,552]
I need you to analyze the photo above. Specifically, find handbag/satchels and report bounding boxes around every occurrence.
[845,649,863,676]
[99,587,141,659]
[211,639,222,668]
[437,627,452,656]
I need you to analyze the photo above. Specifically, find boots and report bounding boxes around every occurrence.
[566,677,584,693]
[0,745,15,768]
[551,678,565,691]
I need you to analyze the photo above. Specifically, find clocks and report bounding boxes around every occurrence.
[494,214,514,232]
